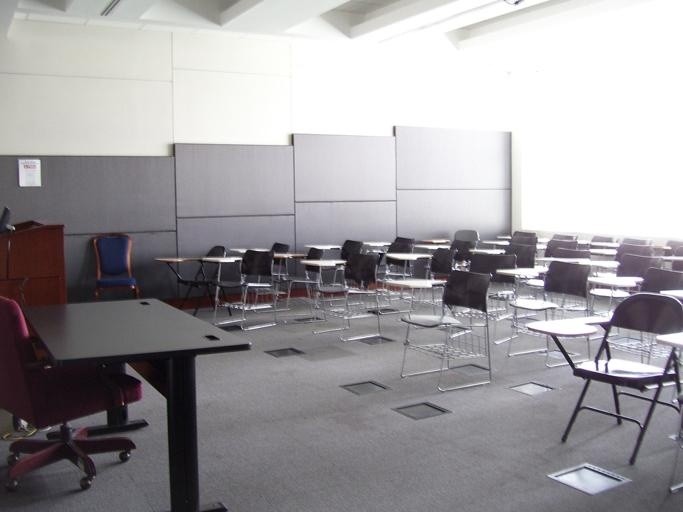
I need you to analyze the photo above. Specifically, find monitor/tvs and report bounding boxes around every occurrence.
[0,206,16,233]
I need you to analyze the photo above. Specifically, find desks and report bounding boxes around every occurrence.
[21,296,254,512]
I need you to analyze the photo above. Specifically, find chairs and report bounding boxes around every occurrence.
[0,296,145,494]
[92,233,140,301]
